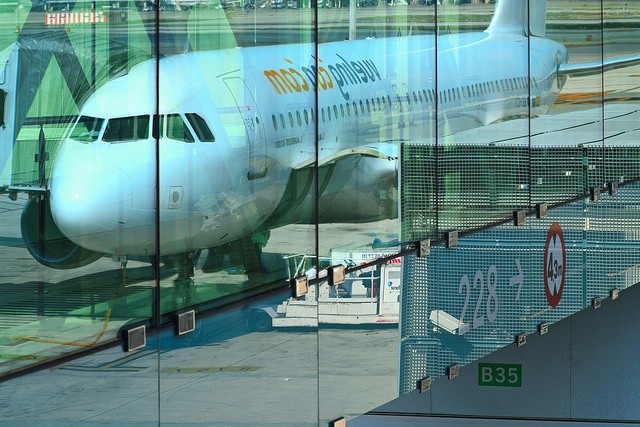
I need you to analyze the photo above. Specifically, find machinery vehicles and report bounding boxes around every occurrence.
[270,247,402,331]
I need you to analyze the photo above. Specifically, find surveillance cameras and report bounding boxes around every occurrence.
[429,309,465,334]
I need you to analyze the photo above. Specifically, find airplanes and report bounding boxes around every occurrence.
[1,1,638,305]
